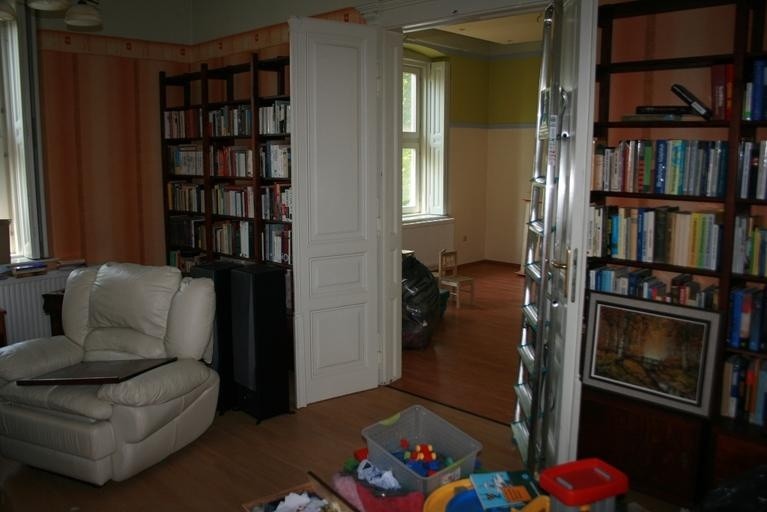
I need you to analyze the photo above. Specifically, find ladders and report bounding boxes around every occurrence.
[510,0,570,473]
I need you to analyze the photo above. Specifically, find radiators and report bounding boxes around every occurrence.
[1,267,74,347]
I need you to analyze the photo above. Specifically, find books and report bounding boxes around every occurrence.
[620,61,766,122]
[589,134,765,200]
[160,101,293,265]
[585,266,766,352]
[716,356,766,426]
[585,204,766,276]
[0,255,87,278]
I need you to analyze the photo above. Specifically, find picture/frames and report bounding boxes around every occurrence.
[575,287,724,421]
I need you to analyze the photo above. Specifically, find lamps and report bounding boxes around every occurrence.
[0,0,106,34]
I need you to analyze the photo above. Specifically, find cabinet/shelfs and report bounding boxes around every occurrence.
[155,56,294,422]
[579,1,766,512]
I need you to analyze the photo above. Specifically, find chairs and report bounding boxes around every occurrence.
[0,257,223,488]
[436,249,475,307]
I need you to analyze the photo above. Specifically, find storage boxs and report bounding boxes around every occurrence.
[541,453,629,512]
[363,403,482,495]
